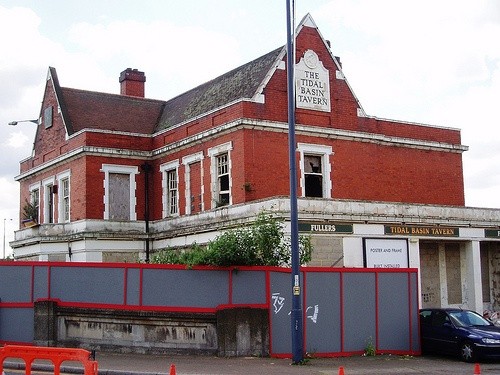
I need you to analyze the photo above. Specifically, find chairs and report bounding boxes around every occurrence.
[420,314,446,325]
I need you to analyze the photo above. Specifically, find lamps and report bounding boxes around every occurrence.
[8,119,40,126]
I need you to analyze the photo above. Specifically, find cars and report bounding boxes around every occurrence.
[420,308,500,364]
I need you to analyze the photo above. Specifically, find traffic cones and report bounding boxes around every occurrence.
[170,364,177,375]
[338,365,346,375]
[474,363,481,375]
